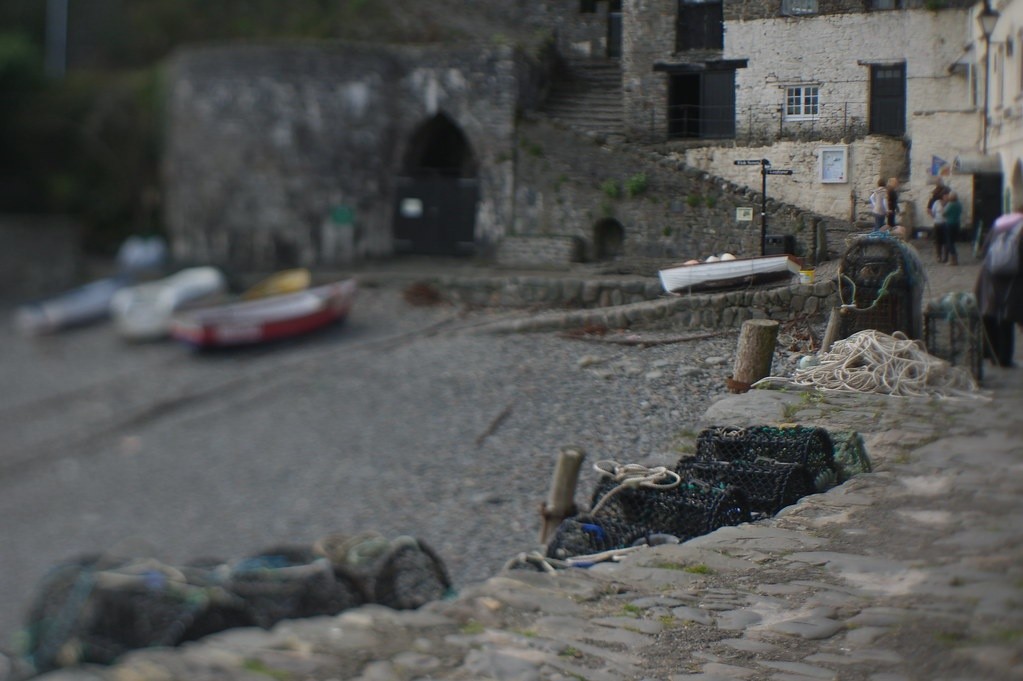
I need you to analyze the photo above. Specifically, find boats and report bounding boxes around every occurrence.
[15,279,117,335]
[660,255,803,295]
[167,267,355,351]
[117,268,226,339]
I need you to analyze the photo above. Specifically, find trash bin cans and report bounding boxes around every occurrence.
[765,234,796,255]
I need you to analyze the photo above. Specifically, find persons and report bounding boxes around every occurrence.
[927,178,963,266]
[975,207,1023,367]
[870,178,900,231]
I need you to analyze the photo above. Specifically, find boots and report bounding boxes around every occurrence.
[952,252,959,265]
[941,246,947,262]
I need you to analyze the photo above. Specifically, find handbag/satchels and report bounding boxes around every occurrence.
[988,213,1023,271]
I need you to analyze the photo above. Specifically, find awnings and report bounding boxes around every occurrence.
[952,156,1001,174]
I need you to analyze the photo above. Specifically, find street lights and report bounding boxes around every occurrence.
[976,0,1002,155]
